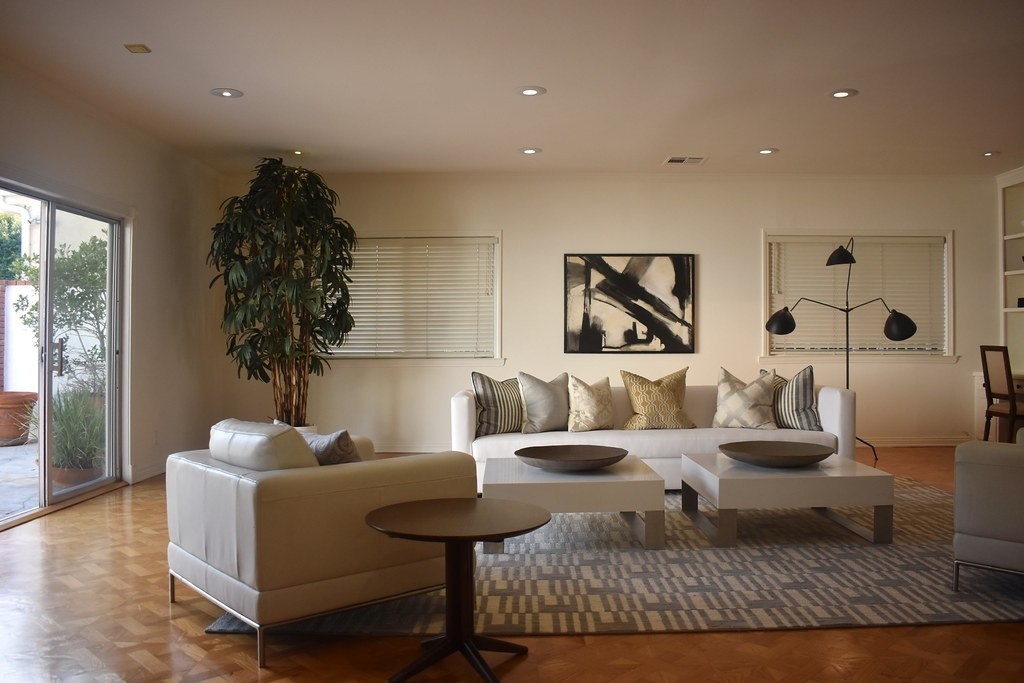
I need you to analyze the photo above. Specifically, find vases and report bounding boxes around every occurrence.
[0,391,40,447]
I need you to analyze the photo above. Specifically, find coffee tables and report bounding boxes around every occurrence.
[481,457,666,554]
[680,454,895,549]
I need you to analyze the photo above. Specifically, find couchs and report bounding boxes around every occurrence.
[451,387,858,495]
[952,426,1024,593]
[165,426,478,669]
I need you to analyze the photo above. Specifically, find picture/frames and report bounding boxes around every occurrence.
[564,254,695,354]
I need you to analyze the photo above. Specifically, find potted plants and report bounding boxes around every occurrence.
[204,156,360,433]
[7,228,107,487]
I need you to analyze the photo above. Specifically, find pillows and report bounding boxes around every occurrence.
[761,365,823,432]
[471,372,523,438]
[566,376,616,432]
[517,371,571,434]
[711,367,777,430]
[620,366,698,430]
[273,419,361,466]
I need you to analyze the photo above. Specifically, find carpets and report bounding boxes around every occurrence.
[204,475,1024,634]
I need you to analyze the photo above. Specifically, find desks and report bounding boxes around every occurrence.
[364,498,552,683]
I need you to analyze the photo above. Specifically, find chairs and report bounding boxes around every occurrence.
[979,345,1024,445]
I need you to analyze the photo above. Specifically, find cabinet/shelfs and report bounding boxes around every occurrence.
[974,369,1024,445]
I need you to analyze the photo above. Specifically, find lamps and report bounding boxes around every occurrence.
[765,237,917,460]
